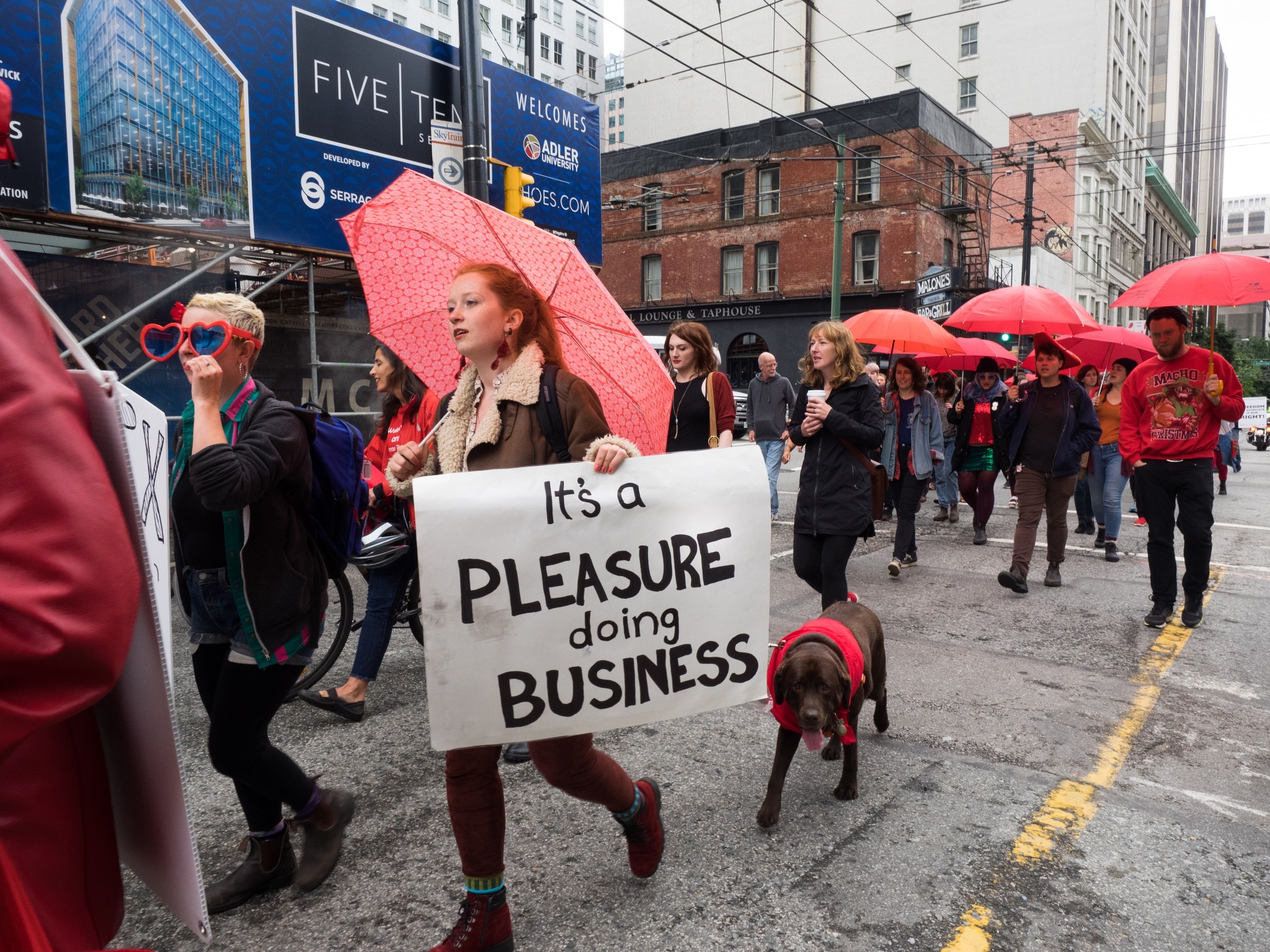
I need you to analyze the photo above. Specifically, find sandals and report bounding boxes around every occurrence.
[296,688,365,720]
[1008,496,1017,508]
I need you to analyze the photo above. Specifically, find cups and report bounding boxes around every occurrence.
[807,389,825,405]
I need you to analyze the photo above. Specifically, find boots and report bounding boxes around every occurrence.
[972,522,987,544]
[1044,561,1062,587]
[998,564,1028,593]
[972,512,978,531]
[204,818,298,915]
[949,503,959,522]
[933,505,948,521]
[285,775,354,891]
[429,886,515,952]
[615,777,666,877]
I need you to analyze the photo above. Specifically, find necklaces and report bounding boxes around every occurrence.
[671,371,697,438]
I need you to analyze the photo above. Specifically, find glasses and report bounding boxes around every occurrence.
[978,375,997,382]
[139,321,261,361]
[761,363,778,368]
[171,331,248,349]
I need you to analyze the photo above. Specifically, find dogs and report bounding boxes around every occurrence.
[758,602,890,827]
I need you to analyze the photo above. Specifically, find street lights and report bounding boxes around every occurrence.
[804,118,846,321]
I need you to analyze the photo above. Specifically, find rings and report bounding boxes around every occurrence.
[813,408,815,413]
[205,362,211,366]
[784,457,788,460]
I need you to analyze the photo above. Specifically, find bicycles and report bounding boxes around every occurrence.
[285,566,424,701]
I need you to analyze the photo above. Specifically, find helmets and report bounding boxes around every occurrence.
[347,496,411,570]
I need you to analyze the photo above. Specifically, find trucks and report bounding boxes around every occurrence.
[643,336,748,440]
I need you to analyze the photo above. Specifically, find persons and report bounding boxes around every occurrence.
[1116,307,1246,629]
[880,354,945,576]
[165,287,358,917]
[746,350,798,522]
[1088,357,1139,563]
[297,339,448,720]
[1211,417,1241,495]
[379,263,668,951]
[0,79,18,198]
[663,320,737,456]
[997,341,1104,594]
[0,231,134,951]
[787,320,888,615]
[856,358,1106,547]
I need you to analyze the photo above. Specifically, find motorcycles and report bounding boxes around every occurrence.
[1246,414,1270,451]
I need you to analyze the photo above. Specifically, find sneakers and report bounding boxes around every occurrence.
[1219,480,1227,495]
[1002,479,1011,488]
[1095,527,1107,547]
[1105,542,1119,562]
[1085,523,1095,534]
[1181,593,1204,628]
[901,551,917,568]
[1128,501,1138,513]
[888,557,902,576]
[1144,597,1173,628]
[1075,523,1087,534]
[1134,517,1147,527]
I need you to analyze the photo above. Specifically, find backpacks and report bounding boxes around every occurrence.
[298,402,368,580]
[867,458,889,521]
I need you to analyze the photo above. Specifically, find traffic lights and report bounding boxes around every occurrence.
[503,166,542,225]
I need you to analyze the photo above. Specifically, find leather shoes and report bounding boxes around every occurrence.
[504,744,530,761]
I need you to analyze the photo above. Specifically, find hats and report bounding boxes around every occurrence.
[975,357,999,374]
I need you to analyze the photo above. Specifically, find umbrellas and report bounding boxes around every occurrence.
[333,167,676,460]
[837,251,1269,387]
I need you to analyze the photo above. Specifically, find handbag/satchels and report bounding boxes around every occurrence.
[1231,439,1237,459]
